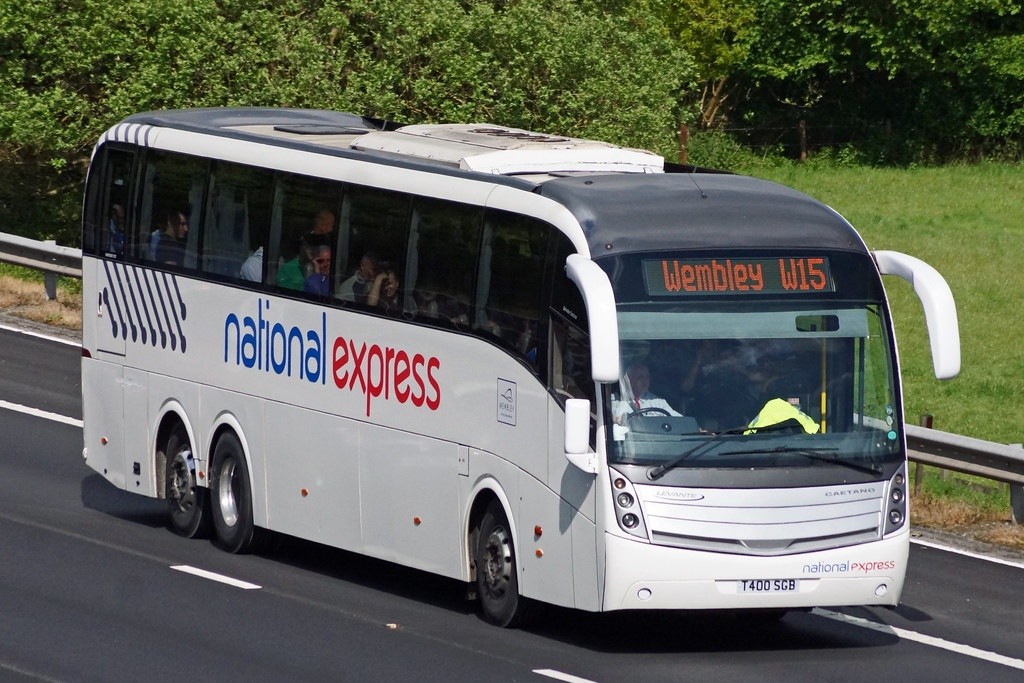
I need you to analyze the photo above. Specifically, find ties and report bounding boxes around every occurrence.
[634,398,641,414]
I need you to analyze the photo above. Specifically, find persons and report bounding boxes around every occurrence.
[107,202,125,253]
[335,241,478,327]
[723,349,807,434]
[154,208,192,268]
[609,362,713,437]
[240,207,334,301]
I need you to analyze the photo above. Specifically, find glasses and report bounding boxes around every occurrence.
[316,258,332,264]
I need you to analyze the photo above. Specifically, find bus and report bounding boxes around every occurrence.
[81,107,961,628]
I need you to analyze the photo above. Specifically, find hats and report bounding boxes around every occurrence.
[312,209,336,235]
